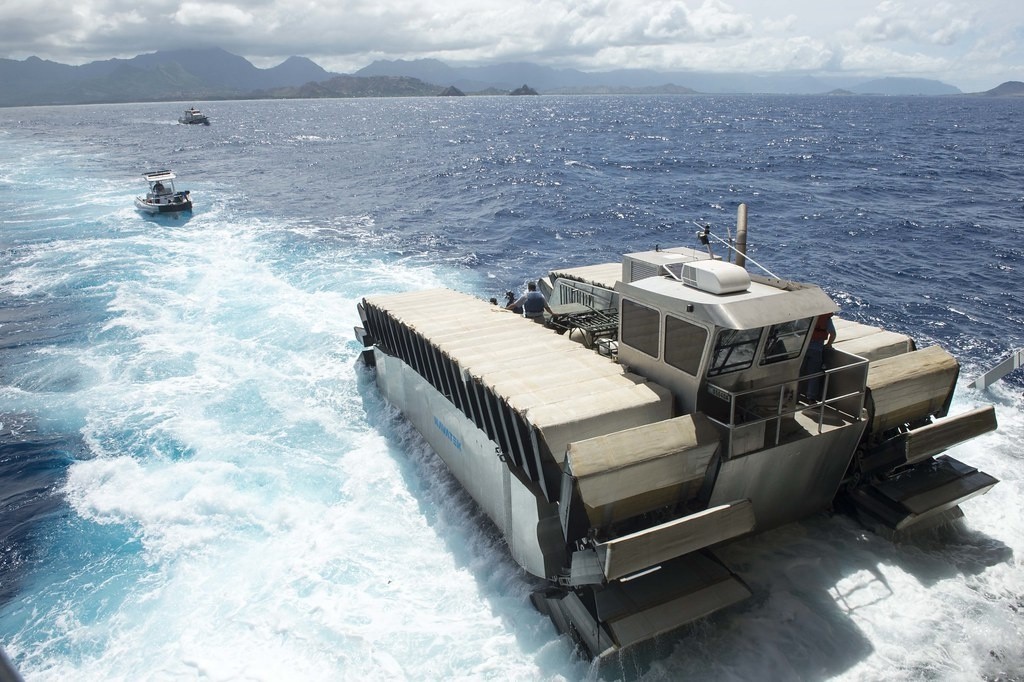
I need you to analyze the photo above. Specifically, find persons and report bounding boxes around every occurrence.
[174,191,190,202]
[506,282,557,324]
[797,312,837,405]
[153,181,164,192]
[506,291,523,314]
[490,298,498,305]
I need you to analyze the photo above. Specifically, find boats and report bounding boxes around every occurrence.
[134,168,193,217]
[352,201,1002,679]
[178,107,210,126]
